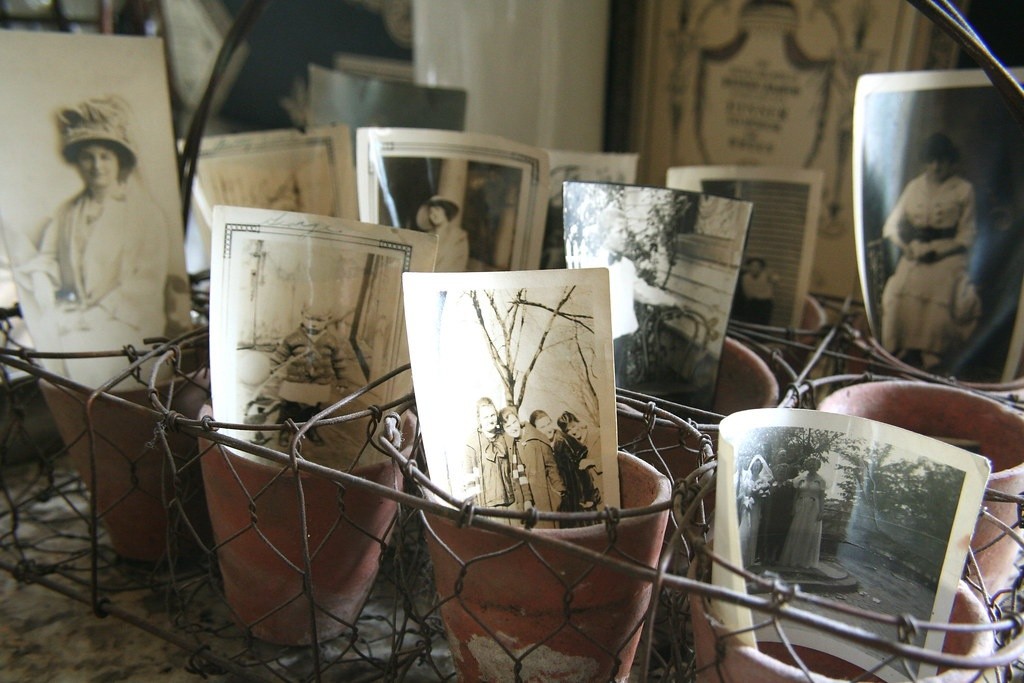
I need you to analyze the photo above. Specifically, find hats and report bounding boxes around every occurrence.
[416,195,459,230]
[921,132,956,157]
[52,93,138,167]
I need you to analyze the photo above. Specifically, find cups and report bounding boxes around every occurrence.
[688,538,994,683]
[617,336,780,484]
[39,368,210,558]
[815,383,1024,606]
[198,395,419,644]
[425,451,672,683]
[762,295,829,349]
[855,314,1024,390]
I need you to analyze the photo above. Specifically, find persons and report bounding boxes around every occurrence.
[471,394,606,532]
[13,94,172,392]
[267,296,357,445]
[874,123,989,370]
[415,191,471,275]
[245,168,298,216]
[736,445,830,576]
[588,205,696,392]
[730,257,790,326]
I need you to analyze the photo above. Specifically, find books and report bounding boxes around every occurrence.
[629,0,970,319]
